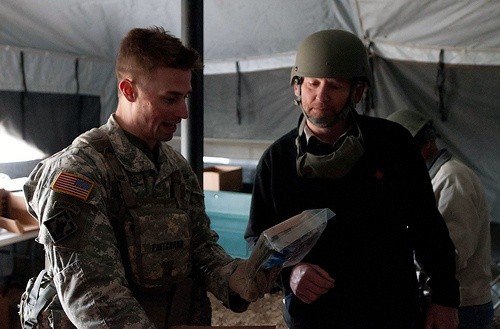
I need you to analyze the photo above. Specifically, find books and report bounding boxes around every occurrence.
[247,207,335,283]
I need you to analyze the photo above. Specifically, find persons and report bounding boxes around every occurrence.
[387,107,494,329]
[23,25,281,329]
[244,30,460,329]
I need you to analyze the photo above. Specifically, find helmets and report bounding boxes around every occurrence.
[385,108,427,138]
[290,30,371,101]
